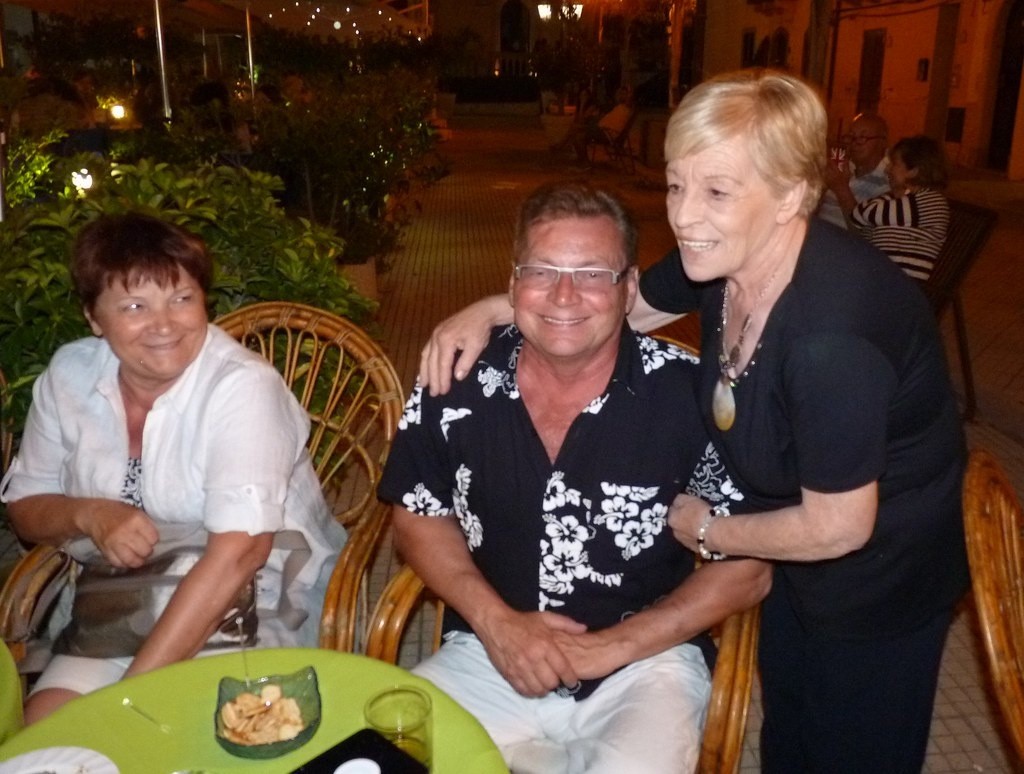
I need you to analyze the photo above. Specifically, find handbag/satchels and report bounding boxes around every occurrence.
[4,545,259,658]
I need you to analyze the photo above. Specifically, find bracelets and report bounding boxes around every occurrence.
[698,506,727,562]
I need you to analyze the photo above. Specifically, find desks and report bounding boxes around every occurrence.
[0,647,519,774]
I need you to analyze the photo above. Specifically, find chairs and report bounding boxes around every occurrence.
[0,304,400,654]
[365,336,757,774]
[923,200,996,408]
[963,454,1024,761]
[588,110,637,175]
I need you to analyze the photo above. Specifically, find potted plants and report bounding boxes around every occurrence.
[279,122,382,306]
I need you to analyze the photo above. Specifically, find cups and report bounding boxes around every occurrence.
[363,686,433,772]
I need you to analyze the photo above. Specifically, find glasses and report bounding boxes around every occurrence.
[513,257,632,291]
[841,133,884,145]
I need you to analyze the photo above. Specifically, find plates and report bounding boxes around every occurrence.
[214,666,321,759]
[290,728,431,774]
[0,747,121,774]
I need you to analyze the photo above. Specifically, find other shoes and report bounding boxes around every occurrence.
[567,162,593,172]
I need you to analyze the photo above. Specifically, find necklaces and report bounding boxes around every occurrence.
[712,214,802,431]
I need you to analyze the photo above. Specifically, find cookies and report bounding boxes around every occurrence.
[220,684,304,746]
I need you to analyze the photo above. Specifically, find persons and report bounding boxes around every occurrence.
[378,68,970,774]
[0,210,360,732]
